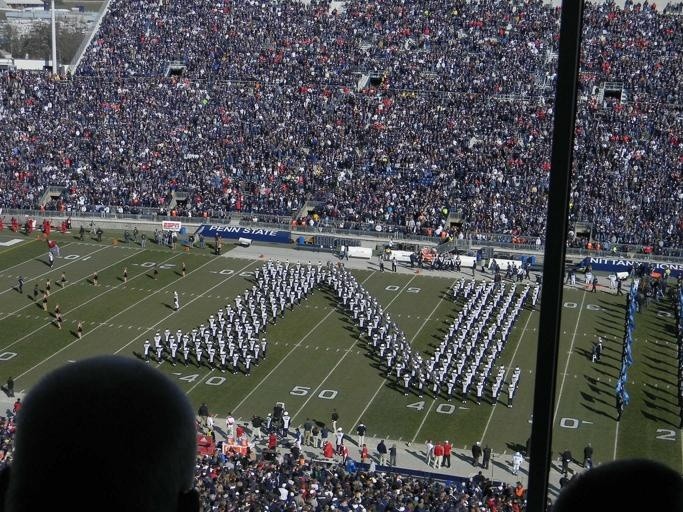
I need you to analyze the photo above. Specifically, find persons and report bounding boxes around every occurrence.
[192,400,596,512]
[0,350,200,512]
[0,397,22,472]
[0,1,682,423]
